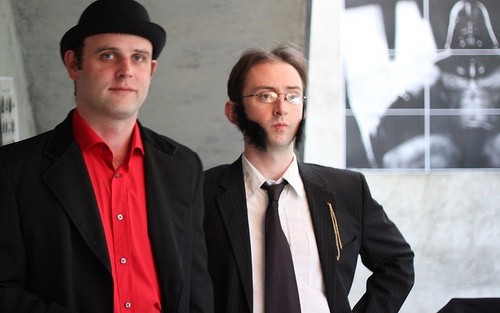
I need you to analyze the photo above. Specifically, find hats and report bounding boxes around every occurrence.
[60,0,166,63]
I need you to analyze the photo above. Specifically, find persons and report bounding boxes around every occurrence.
[204,42,415,313]
[0,0,217,313]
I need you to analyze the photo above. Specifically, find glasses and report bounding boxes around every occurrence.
[242,91,306,104]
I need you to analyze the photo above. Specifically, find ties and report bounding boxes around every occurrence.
[260,180,301,313]
